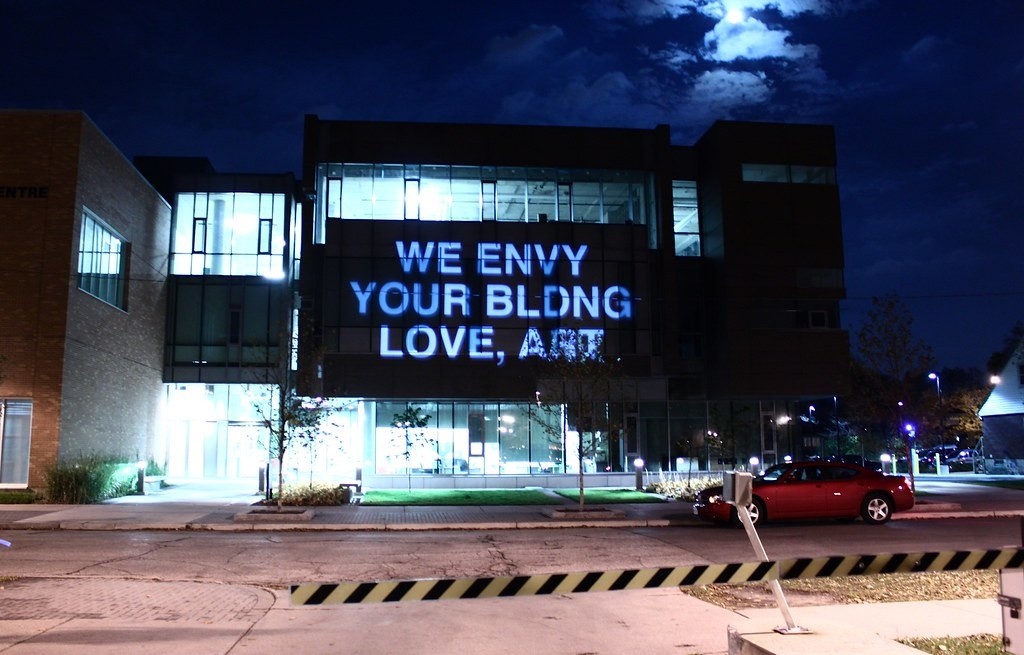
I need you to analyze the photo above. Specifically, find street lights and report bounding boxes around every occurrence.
[809,404,816,448]
[929,372,946,464]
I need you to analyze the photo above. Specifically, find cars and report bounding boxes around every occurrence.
[899,443,961,465]
[942,447,982,472]
[801,454,883,480]
[692,461,915,530]
